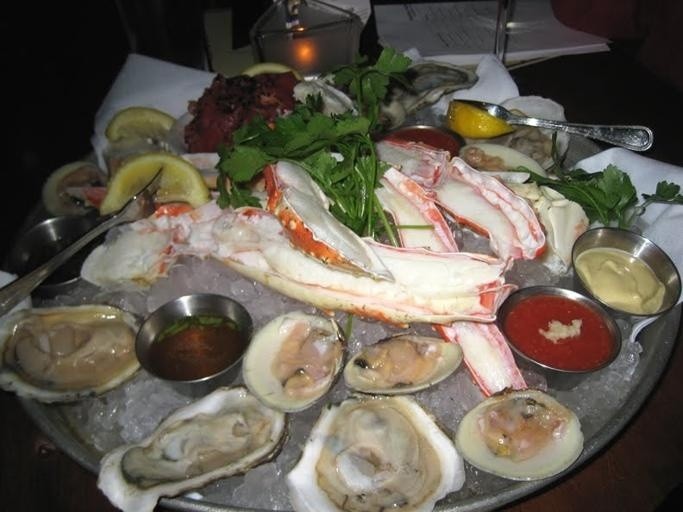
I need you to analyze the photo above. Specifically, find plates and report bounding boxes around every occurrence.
[0,128,683,510]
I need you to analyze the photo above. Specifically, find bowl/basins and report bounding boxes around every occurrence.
[383,125,462,156]
[570,225,682,322]
[133,293,253,396]
[498,284,623,392]
[9,213,116,299]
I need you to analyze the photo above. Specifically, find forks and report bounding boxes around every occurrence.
[0,169,163,316]
[452,98,653,155]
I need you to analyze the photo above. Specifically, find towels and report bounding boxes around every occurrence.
[568,138,681,306]
[89,47,220,179]
[1,267,33,395]
[360,47,525,117]
